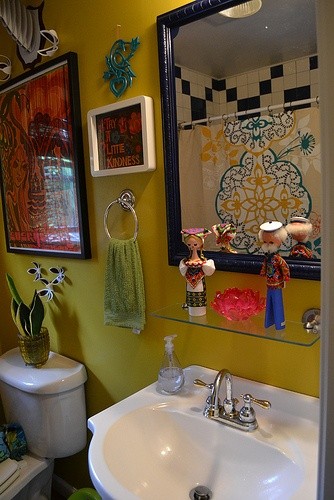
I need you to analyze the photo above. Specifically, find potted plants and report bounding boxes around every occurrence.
[5,273,49,369]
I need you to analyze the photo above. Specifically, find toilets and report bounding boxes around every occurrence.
[0,345,88,500]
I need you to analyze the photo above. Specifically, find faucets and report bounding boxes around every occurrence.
[193,369,272,432]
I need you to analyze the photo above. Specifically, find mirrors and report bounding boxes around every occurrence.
[156,0,322,281]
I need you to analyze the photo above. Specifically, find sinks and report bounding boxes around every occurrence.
[88,365,321,500]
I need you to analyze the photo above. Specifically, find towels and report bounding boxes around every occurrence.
[104,237,147,335]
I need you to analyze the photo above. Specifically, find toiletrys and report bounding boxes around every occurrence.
[158,334,186,395]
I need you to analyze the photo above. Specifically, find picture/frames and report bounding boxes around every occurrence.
[86,95,155,177]
[0,50,93,261]
